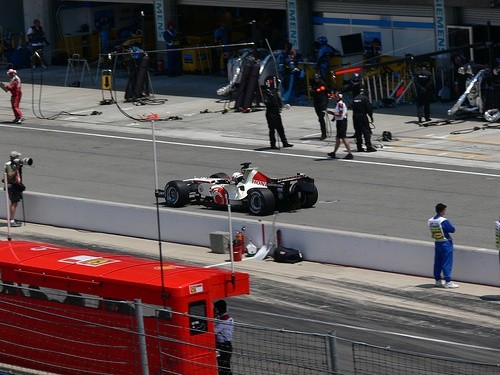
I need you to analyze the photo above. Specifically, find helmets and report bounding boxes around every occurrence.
[231,172,243,182]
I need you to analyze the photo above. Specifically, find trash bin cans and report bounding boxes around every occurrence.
[228,242,243,261]
[209,231,229,254]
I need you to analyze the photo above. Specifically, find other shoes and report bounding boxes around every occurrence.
[271,146,279,150]
[357,148,364,152]
[33,65,36,70]
[426,118,431,121]
[435,280,445,286]
[444,281,459,288]
[283,144,293,147]
[320,135,327,140]
[367,148,377,152]
[41,64,47,69]
[419,118,422,123]
[343,153,353,160]
[327,152,335,159]
[13,117,25,124]
[10,219,22,227]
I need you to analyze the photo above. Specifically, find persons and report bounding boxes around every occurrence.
[309,73,329,140]
[2,151,33,227]
[367,36,382,65]
[121,46,155,102]
[213,299,233,375]
[353,88,377,152]
[0,69,24,124]
[327,93,353,159]
[428,203,459,288]
[265,89,293,149]
[26,19,48,69]
[454,56,466,107]
[316,37,335,81]
[213,26,233,59]
[163,21,182,77]
[286,49,303,75]
[414,61,433,123]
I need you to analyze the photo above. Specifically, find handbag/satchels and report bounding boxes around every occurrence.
[273,245,302,264]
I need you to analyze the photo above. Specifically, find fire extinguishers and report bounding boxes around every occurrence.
[235,226,245,254]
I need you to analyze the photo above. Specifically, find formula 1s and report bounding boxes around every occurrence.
[153,161,319,217]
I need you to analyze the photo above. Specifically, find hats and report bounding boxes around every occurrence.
[317,36,328,42]
[7,69,17,75]
[10,151,21,157]
[436,203,447,212]
